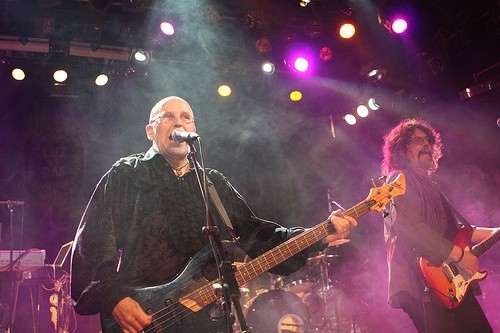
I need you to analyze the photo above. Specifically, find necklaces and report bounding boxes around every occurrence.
[172,161,189,175]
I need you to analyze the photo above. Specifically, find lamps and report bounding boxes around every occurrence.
[10,10,413,125]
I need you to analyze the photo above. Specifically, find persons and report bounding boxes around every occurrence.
[69,96,357,333]
[379,119,500,333]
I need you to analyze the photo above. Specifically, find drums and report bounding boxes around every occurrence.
[232,289,310,333]
[285,282,324,314]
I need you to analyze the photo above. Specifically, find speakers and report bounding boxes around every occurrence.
[0,265,103,333]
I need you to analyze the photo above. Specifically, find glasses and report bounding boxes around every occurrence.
[150,113,195,124]
[409,136,430,145]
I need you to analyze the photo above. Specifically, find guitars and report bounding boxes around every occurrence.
[416,229,500,312]
[100,172,406,333]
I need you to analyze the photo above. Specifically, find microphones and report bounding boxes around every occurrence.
[327,191,332,213]
[171,130,199,143]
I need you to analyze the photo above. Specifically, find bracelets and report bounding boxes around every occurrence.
[453,248,464,263]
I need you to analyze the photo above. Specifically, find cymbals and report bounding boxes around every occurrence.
[307,254,343,261]
[327,239,350,247]
